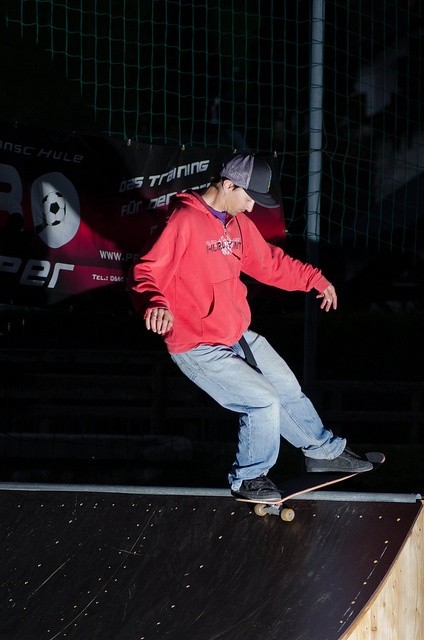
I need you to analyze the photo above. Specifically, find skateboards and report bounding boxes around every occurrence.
[233,451,385,522]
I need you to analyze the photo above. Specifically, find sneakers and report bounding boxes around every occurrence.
[231,475,282,502]
[305,447,374,473]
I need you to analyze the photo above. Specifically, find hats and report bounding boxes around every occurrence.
[220,153,280,209]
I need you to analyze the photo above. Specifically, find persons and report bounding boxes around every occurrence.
[126,152,373,504]
